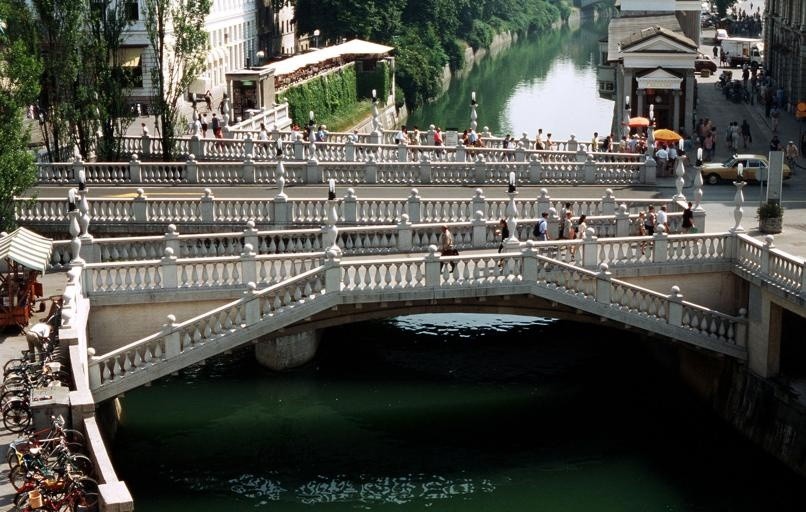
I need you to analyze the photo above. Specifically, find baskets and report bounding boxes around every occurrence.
[29,491,43,509]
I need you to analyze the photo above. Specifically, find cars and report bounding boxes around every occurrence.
[699,152,792,185]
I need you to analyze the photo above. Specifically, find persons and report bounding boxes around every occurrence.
[37,111,46,130]
[498,220,509,253]
[191,89,231,138]
[637,204,668,254]
[256,123,270,156]
[439,224,456,274]
[732,177,747,211]
[535,211,551,241]
[353,129,362,153]
[561,203,588,239]
[138,121,149,139]
[288,120,332,154]
[680,201,699,234]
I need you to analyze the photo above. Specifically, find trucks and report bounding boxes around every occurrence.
[720,37,765,70]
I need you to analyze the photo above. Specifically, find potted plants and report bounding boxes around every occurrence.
[754,200,786,235]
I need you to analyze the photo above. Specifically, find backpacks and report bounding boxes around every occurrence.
[534,221,544,236]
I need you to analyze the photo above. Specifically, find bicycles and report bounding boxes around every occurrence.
[0,316,102,512]
